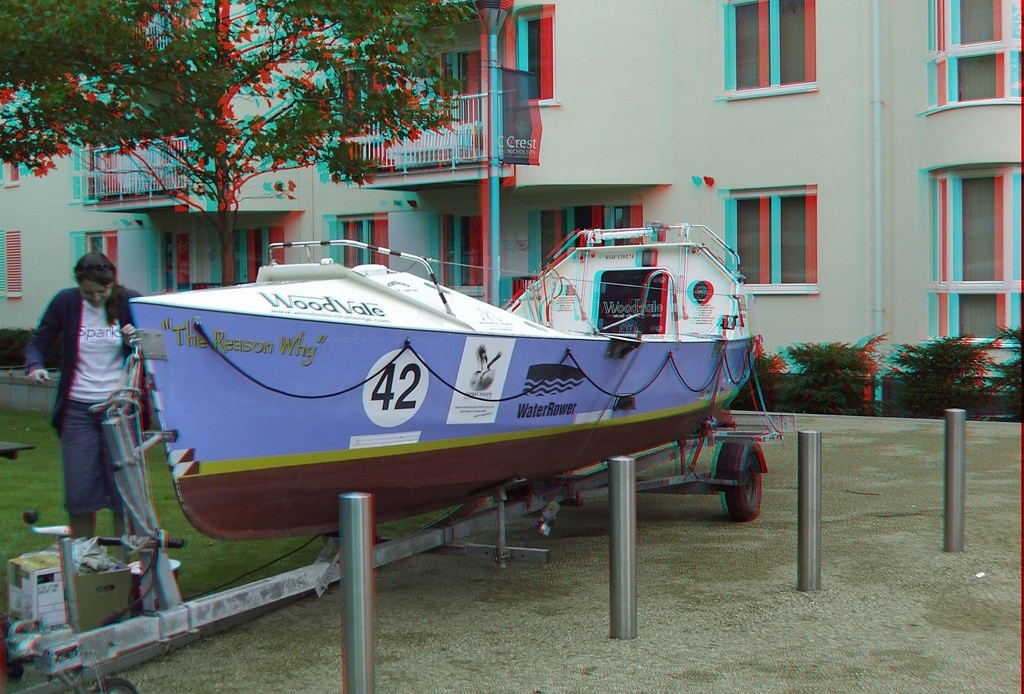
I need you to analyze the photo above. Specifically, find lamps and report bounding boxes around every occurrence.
[516,231,528,250]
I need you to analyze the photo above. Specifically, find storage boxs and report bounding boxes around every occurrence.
[77,557,132,632]
[6,552,67,627]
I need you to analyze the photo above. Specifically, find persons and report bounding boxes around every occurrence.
[24,252,152,563]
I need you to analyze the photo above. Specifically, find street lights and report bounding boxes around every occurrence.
[476,0,511,304]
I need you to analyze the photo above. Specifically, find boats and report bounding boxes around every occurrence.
[128,224,757,540]
